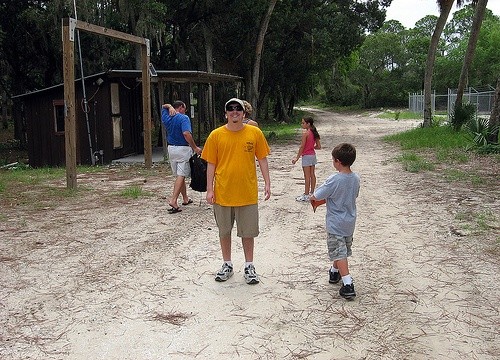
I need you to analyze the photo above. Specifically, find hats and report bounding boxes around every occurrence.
[225,98,245,111]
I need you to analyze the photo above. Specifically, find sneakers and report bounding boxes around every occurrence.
[215,262,234,282]
[243,264,259,284]
[328,266,342,283]
[339,283,356,298]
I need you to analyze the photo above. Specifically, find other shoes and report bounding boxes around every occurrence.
[296,191,315,202]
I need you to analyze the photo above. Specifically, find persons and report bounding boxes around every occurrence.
[161,101,203,212]
[291,116,322,202]
[199,98,271,284]
[241,100,259,126]
[309,143,360,298]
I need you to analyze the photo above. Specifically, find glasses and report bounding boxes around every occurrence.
[227,105,243,111]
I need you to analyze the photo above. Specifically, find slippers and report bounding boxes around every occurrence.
[182,198,194,205]
[167,203,182,212]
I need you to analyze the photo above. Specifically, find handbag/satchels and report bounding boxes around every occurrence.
[189,153,208,192]
